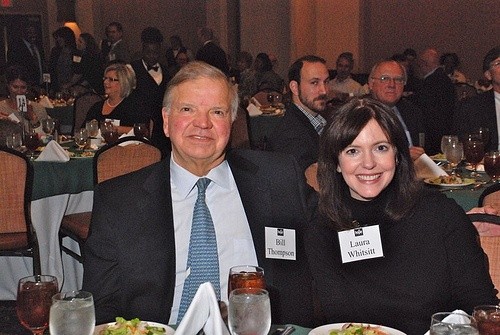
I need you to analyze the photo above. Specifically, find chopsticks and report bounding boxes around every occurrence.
[437,162,445,167]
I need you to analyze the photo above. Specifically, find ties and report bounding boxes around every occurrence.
[30,45,40,80]
[176,178,221,323]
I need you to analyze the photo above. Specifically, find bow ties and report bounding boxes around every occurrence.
[147,66,159,72]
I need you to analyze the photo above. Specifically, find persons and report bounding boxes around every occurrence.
[0,22,358,112]
[78,62,321,329]
[454,47,500,154]
[81,64,151,138]
[0,73,48,138]
[329,47,467,158]
[358,60,428,162]
[269,54,334,161]
[298,96,499,335]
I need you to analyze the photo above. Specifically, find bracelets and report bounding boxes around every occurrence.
[30,119,41,130]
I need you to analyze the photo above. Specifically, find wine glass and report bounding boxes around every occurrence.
[445,141,463,175]
[267,91,281,108]
[484,152,500,183]
[25,132,38,160]
[463,140,485,178]
[74,129,88,150]
[17,275,59,335]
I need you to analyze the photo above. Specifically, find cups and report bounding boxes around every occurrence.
[441,136,458,153]
[49,291,96,335]
[431,305,500,335]
[134,123,144,137]
[42,118,52,133]
[227,265,271,335]
[57,92,77,99]
[465,127,489,147]
[86,120,118,144]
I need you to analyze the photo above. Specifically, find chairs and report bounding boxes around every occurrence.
[59,136,164,265]
[231,89,500,295]
[0,144,43,279]
[65,84,104,134]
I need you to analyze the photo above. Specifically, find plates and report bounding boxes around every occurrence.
[424,178,473,186]
[465,164,486,173]
[93,321,176,335]
[308,323,406,335]
[261,108,281,115]
[434,159,447,162]
[55,102,74,106]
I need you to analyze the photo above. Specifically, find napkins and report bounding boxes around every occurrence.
[423,308,469,335]
[174,280,229,335]
[34,140,70,162]
[412,154,448,179]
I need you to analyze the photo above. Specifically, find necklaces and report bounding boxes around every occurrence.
[105,95,125,107]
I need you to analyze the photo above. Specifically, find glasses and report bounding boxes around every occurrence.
[102,76,119,83]
[371,77,405,84]
[488,61,500,69]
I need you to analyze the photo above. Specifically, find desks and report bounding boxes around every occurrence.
[0,140,95,300]
[418,153,496,211]
[45,105,75,136]
